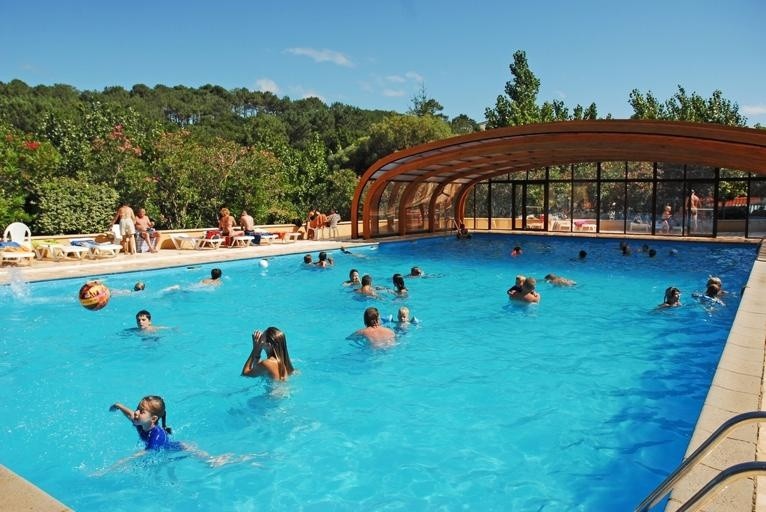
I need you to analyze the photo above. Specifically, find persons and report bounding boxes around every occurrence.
[393,305,411,328]
[388,273,408,297]
[240,327,293,409]
[505,275,525,296]
[218,207,244,246]
[630,187,703,233]
[576,249,588,260]
[705,276,730,298]
[312,252,334,269]
[509,277,540,303]
[302,253,312,265]
[239,210,255,246]
[353,274,379,297]
[544,274,577,287]
[305,206,341,241]
[91,395,249,476]
[134,309,156,330]
[354,307,395,345]
[698,285,723,307]
[201,267,222,285]
[340,246,356,255]
[660,286,682,308]
[617,241,678,257]
[511,246,523,256]
[109,201,136,258]
[410,266,421,278]
[131,206,161,253]
[341,269,361,288]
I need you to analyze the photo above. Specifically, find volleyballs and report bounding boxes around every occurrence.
[80,281,110,311]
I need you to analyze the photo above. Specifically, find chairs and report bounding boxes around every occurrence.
[517,213,601,231]
[630,220,698,233]
